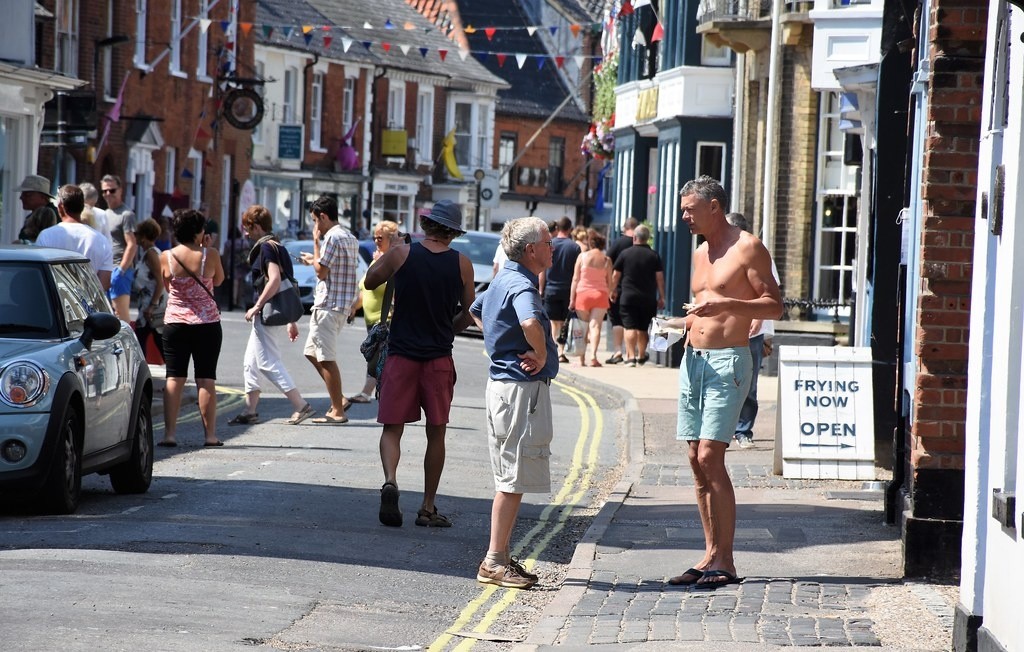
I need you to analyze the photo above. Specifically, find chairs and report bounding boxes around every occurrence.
[10,272,51,328]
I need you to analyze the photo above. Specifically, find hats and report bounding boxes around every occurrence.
[420,200,467,233]
[12,175,56,200]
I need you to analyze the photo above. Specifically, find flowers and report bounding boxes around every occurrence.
[580,113,616,160]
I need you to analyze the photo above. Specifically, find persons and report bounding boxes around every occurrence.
[227,205,316,425]
[79,183,112,239]
[364,201,475,528]
[726,212,781,448]
[492,243,508,276]
[299,196,359,423]
[35,186,113,291]
[199,203,219,246]
[101,174,138,324]
[154,218,172,252]
[668,175,784,589]
[469,217,559,587]
[11,175,57,245]
[132,218,168,364]
[538,216,665,367]
[346,220,399,403]
[157,208,224,447]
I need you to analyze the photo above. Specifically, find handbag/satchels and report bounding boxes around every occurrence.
[149,311,166,334]
[253,240,304,326]
[368,351,379,379]
[556,309,589,357]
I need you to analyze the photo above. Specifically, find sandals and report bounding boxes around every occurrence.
[349,393,371,403]
[228,413,259,425]
[379,481,402,527]
[283,403,317,425]
[415,505,451,527]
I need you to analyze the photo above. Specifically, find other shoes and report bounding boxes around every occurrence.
[558,354,649,367]
[736,438,755,449]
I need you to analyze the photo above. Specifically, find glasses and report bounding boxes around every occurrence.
[100,189,116,194]
[373,236,389,242]
[312,201,321,211]
[524,240,552,251]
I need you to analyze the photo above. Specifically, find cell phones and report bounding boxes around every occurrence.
[289,252,307,262]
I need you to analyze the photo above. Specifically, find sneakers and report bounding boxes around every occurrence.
[509,555,538,583]
[477,555,534,588]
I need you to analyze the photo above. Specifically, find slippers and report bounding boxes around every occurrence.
[157,442,177,447]
[328,399,352,413]
[695,571,743,589]
[204,440,223,446]
[669,568,704,585]
[312,416,348,423]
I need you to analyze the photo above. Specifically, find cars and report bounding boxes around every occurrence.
[0,243,156,509]
[281,232,425,317]
[448,230,502,337]
